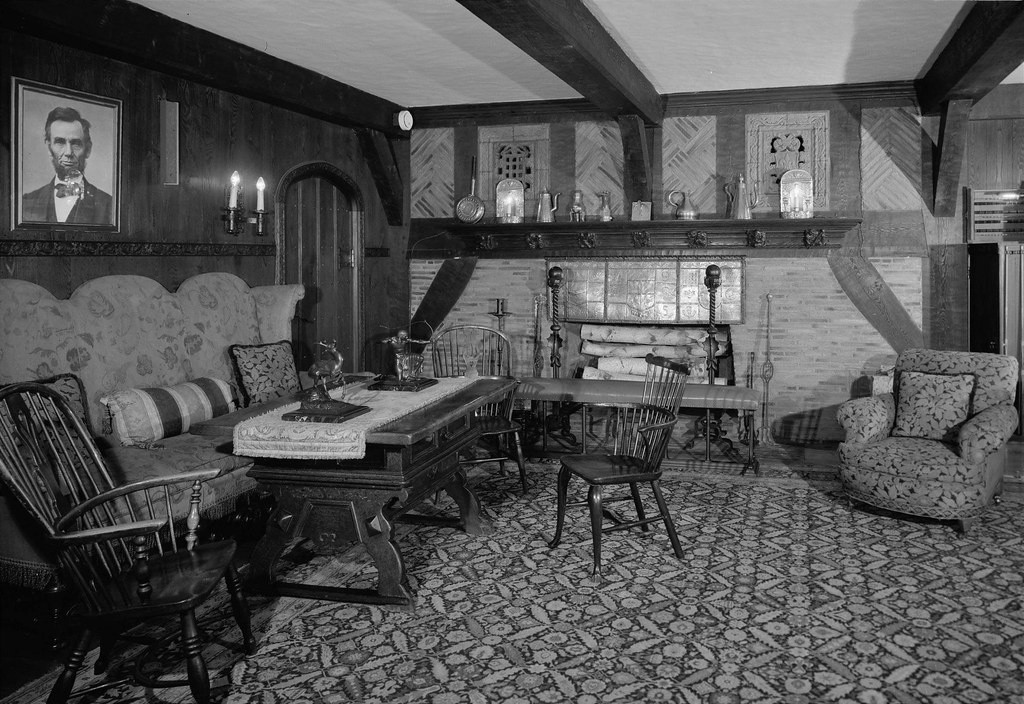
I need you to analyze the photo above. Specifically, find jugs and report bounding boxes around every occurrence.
[667,190,701,219]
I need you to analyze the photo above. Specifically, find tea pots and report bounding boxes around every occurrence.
[723,173,760,219]
[535,185,560,222]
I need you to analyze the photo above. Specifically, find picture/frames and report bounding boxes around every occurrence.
[9,76,124,234]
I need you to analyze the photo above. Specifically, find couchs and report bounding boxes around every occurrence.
[836,347,1019,532]
[0,272,374,659]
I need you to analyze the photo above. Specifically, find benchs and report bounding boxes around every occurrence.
[515,377,762,475]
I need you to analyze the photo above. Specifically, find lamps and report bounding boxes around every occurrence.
[220,171,244,234]
[249,177,271,236]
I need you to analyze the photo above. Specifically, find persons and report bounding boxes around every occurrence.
[383,329,435,382]
[21,107,112,226]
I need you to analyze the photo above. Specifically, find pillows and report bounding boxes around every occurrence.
[229,340,304,409]
[1,373,97,493]
[890,370,978,443]
[100,377,236,446]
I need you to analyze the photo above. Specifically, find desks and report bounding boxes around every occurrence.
[189,374,516,613]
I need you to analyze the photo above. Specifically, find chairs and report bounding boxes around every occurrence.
[0,382,257,704]
[548,353,691,582]
[432,325,529,505]
